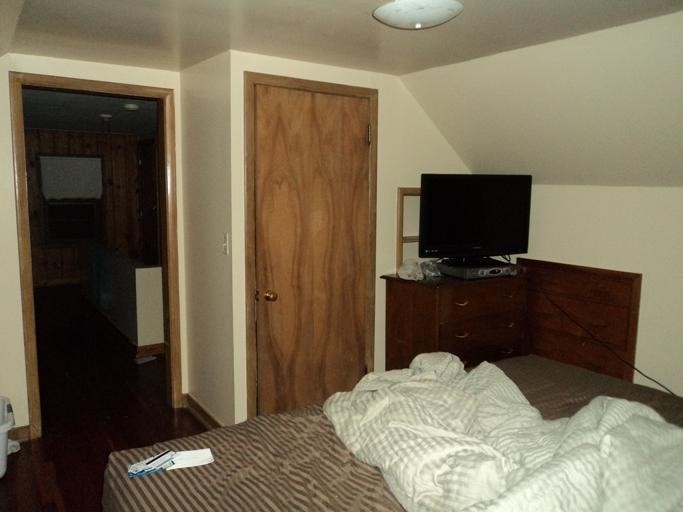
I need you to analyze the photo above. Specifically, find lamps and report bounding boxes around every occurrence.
[373,0,465,30]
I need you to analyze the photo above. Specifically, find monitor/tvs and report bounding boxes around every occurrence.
[418,173,531,268]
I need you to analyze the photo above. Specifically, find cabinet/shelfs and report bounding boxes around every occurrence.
[80,243,165,358]
[380,265,530,371]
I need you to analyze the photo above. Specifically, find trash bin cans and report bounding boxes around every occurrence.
[0,395,15,478]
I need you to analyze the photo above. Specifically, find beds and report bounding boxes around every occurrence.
[101,351,683,512]
